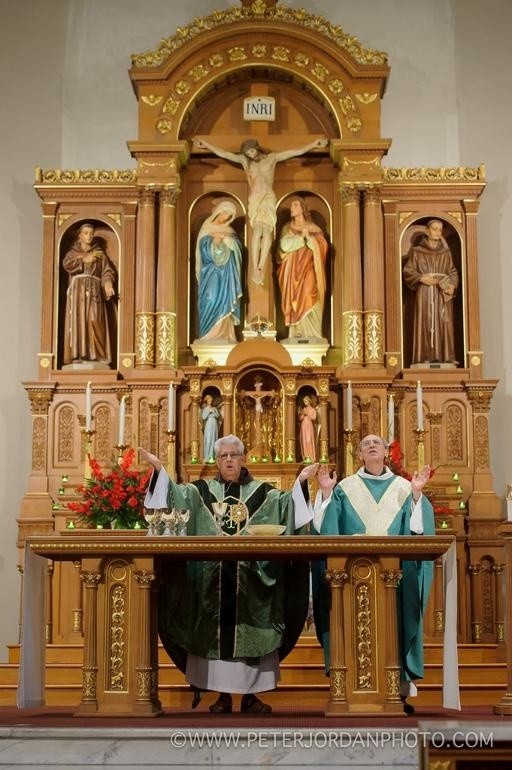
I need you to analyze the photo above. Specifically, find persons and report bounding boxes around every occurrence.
[194,201,244,343]
[403,219,459,364]
[62,223,118,365]
[194,137,328,285]
[275,198,328,340]
[298,396,317,463]
[248,393,269,416]
[202,395,221,459]
[135,434,319,715]
[310,435,436,714]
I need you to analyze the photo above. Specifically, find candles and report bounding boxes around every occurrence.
[346,380,353,427]
[86,381,92,429]
[119,393,129,444]
[417,380,423,429]
[169,381,177,430]
[388,392,394,444]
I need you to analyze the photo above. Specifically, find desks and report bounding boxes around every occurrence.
[26,534,454,716]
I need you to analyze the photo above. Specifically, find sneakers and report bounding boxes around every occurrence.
[209,694,232,713]
[241,695,272,713]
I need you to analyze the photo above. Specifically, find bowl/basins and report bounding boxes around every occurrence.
[244,525,287,536]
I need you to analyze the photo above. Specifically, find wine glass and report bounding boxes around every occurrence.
[143,507,191,538]
[212,502,228,535]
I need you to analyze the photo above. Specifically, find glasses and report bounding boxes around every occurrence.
[218,453,242,461]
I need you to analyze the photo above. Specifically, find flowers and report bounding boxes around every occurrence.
[383,440,454,515]
[70,447,153,529]
